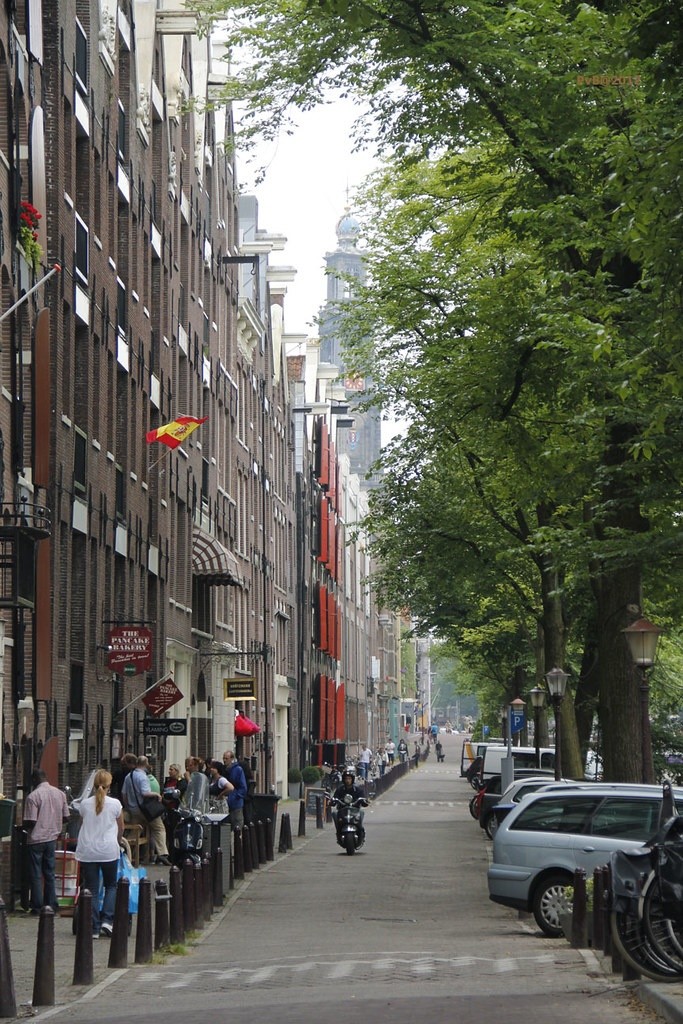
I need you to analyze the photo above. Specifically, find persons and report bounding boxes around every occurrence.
[358,744,372,781]
[375,747,389,776]
[412,741,420,766]
[74,770,124,938]
[121,750,255,866]
[330,770,370,842]
[435,741,442,755]
[22,768,71,914]
[397,739,408,762]
[430,722,438,742]
[385,739,396,765]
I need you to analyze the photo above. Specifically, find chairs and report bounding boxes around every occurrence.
[124,822,150,868]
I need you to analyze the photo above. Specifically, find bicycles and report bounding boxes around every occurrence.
[609,815,683,983]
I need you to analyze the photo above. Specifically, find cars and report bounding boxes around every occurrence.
[490,777,576,841]
[476,768,555,840]
[459,740,604,819]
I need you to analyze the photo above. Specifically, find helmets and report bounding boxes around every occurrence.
[342,769,355,785]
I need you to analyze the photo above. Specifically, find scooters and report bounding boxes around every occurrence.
[64,785,90,852]
[324,787,376,855]
[172,789,216,870]
[320,755,380,793]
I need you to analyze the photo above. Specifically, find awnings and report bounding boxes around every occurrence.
[193,528,244,587]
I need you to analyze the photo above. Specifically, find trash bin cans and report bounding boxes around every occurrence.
[255,794,283,849]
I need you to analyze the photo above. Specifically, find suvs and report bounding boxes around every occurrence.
[489,782,683,937]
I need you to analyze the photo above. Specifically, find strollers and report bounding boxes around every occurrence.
[73,837,147,937]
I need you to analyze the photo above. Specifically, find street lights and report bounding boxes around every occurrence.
[528,686,547,769]
[620,617,664,785]
[544,663,572,781]
[499,705,508,746]
[510,696,527,748]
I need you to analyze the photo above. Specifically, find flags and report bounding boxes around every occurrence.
[145,416,206,447]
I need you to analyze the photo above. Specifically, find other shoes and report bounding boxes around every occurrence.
[28,909,42,919]
[92,932,101,939]
[156,855,172,866]
[102,923,112,937]
[53,912,61,920]
[150,855,158,865]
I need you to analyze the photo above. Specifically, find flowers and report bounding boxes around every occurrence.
[18,200,44,270]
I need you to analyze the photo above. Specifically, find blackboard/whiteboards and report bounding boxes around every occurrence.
[305,787,327,818]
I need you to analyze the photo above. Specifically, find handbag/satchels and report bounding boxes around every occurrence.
[382,761,386,766]
[96,851,147,915]
[609,817,683,922]
[140,797,166,822]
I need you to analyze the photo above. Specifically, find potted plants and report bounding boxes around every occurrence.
[302,765,320,798]
[288,767,302,800]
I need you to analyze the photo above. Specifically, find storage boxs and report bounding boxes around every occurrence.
[55,849,78,906]
